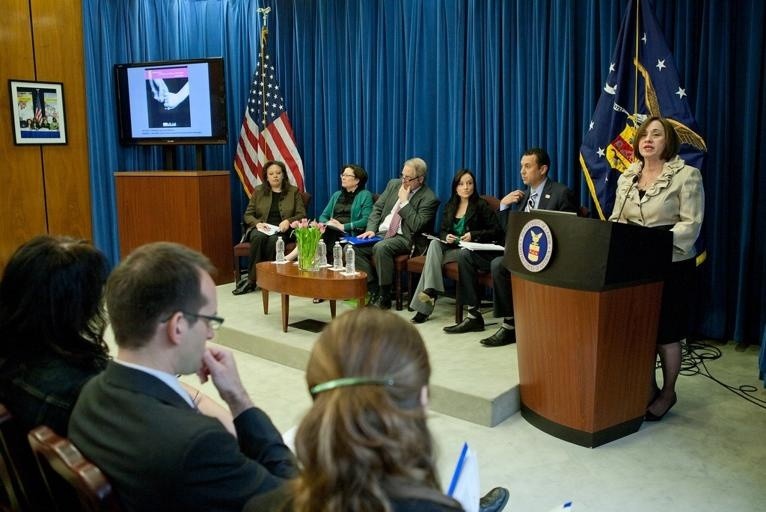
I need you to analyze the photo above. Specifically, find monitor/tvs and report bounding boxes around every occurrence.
[113,56,229,146]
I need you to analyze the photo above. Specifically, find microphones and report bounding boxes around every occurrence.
[615,175,638,222]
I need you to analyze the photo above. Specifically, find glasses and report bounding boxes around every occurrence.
[161,309,224,331]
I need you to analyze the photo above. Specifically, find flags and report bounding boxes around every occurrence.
[234,24,305,204]
[578,0,708,222]
[33,88,42,127]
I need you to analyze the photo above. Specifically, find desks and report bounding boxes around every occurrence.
[254,260,368,333]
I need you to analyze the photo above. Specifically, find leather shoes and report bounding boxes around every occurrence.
[313,298,324,303]
[478,486,510,512]
[443,316,485,334]
[643,387,678,422]
[479,326,517,347]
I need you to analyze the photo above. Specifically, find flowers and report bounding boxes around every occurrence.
[289,219,327,267]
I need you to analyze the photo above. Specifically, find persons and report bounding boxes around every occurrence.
[408,168,507,323]
[242,303,466,512]
[443,148,582,347]
[355,157,442,311]
[161,80,189,110]
[17,99,59,130]
[66,240,510,512]
[230,158,308,296]
[606,115,706,423]
[0,233,112,444]
[312,163,373,304]
[148,70,169,104]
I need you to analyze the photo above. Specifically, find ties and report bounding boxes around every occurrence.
[523,191,538,213]
[384,201,402,240]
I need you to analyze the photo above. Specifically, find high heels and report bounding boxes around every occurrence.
[231,276,257,295]
[410,312,430,324]
[418,288,438,306]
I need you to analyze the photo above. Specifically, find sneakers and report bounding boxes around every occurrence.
[368,291,392,310]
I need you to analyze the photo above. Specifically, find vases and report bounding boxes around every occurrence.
[299,243,320,272]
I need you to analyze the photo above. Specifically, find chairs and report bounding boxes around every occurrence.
[25,420,113,509]
[322,193,378,250]
[0,378,46,511]
[405,193,502,324]
[474,205,589,297]
[373,199,440,313]
[233,191,313,285]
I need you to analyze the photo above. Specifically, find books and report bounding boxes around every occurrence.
[456,241,507,252]
[340,236,384,244]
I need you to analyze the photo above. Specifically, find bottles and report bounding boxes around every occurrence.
[332,241,343,269]
[318,238,327,266]
[275,236,284,262]
[345,244,355,274]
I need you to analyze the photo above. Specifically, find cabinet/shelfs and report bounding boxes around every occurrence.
[114,169,239,287]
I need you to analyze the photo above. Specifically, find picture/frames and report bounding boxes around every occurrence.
[6,80,70,146]
